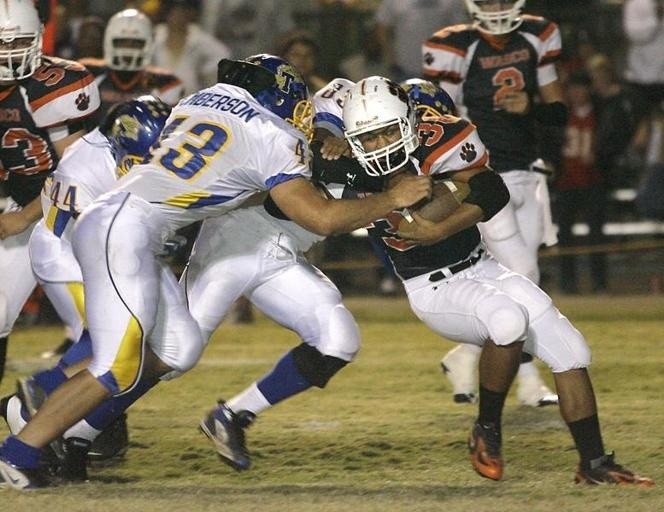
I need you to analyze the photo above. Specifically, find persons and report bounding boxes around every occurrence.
[263,76,655,488]
[587,53,642,159]
[51,78,459,487]
[80,9,184,126]
[287,36,330,90]
[0,52,433,492]
[0,0,100,388]
[421,1,566,407]
[151,1,231,92]
[543,72,618,292]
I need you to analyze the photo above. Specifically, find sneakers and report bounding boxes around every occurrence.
[514,375,558,407]
[0,453,59,489]
[574,453,653,489]
[201,400,256,470]
[88,412,133,467]
[18,376,68,460]
[442,344,481,403]
[468,422,503,481]
[0,394,71,484]
[59,434,92,483]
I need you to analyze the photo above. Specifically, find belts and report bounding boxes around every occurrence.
[95,193,181,256]
[404,248,485,293]
[207,206,306,263]
[490,157,552,179]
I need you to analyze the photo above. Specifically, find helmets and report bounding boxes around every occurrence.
[105,9,152,70]
[0,0,45,81]
[343,75,419,177]
[398,77,458,117]
[217,53,314,143]
[103,94,174,178]
[467,0,525,35]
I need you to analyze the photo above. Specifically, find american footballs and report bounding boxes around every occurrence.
[397,180,472,235]
